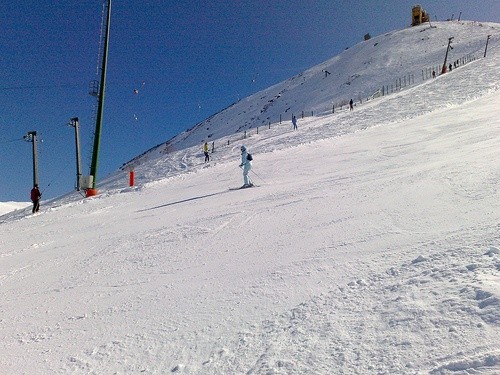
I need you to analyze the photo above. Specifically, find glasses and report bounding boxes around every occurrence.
[241,149,243,151]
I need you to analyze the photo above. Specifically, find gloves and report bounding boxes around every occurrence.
[240,163,244,166]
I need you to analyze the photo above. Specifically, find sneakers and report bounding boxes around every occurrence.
[242,184,249,187]
[249,183,253,187]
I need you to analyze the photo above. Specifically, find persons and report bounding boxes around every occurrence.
[292,116,297,130]
[204,143,210,163]
[240,146,252,187]
[29,183,42,213]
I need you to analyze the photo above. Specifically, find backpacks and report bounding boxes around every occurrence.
[247,154,253,161]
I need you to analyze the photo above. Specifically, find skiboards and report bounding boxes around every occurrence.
[229,185,261,190]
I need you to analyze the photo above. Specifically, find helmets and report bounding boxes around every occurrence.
[35,184,39,189]
[241,146,246,151]
